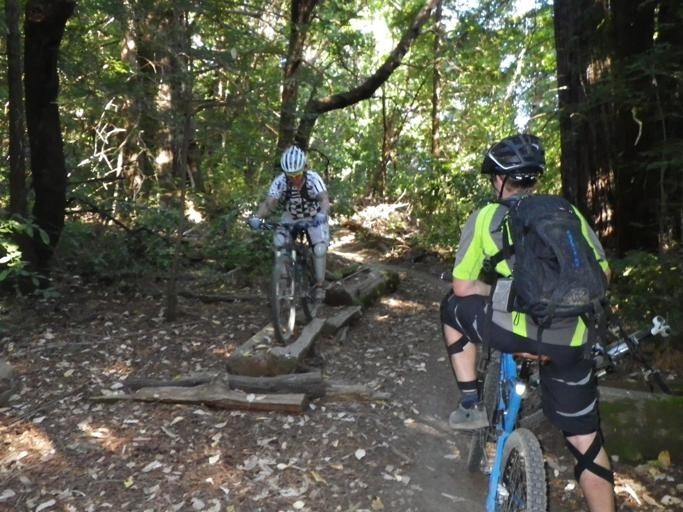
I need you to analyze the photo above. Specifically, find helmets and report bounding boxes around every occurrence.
[482,134,546,175]
[280,146,305,177]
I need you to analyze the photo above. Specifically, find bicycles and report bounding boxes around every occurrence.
[482,293,680,436]
[247,209,323,347]
[436,262,555,512]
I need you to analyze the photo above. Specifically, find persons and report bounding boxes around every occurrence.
[248,146,332,301]
[436,132,620,512]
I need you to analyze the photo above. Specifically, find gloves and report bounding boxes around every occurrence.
[314,215,326,224]
[250,217,260,228]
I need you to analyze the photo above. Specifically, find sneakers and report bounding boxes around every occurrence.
[316,288,326,302]
[449,403,489,430]
[279,276,288,287]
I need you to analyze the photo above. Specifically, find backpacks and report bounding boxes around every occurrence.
[484,195,608,378]
